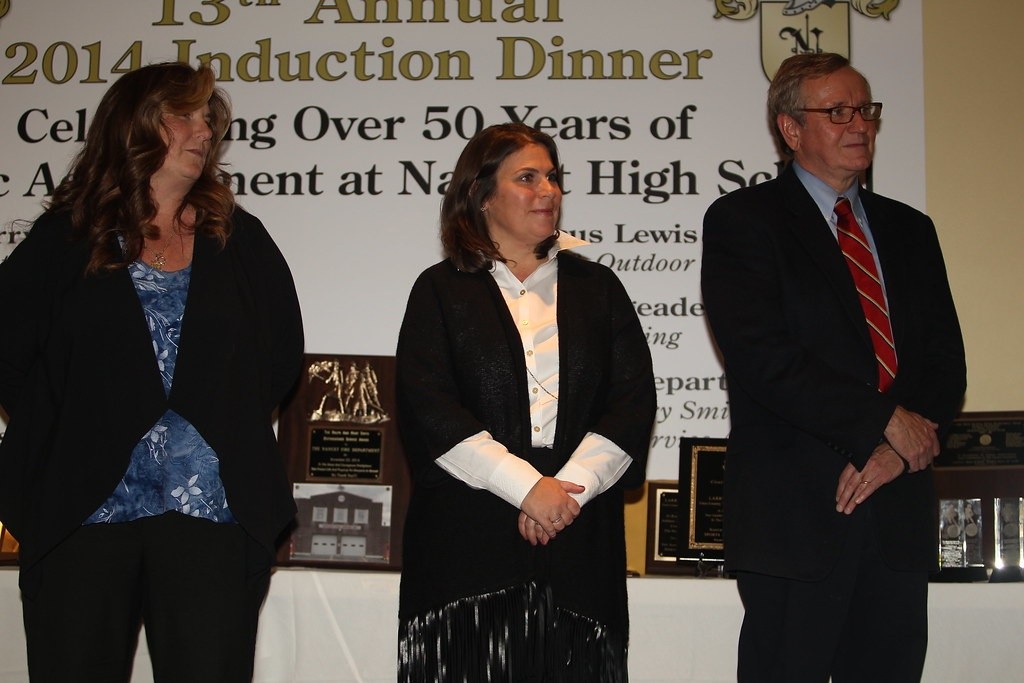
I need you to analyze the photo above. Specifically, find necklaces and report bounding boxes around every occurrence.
[145,222,179,269]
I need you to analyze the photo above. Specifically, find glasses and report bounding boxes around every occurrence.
[792,100,884,123]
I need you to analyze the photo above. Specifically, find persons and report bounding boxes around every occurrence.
[395,123,657,683]
[317,362,383,420]
[0,63,306,683]
[700,52,969,683]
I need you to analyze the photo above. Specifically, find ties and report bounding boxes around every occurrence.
[832,196,899,407]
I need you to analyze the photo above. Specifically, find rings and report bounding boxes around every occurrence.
[860,481,871,484]
[535,523,540,525]
[551,516,562,523]
